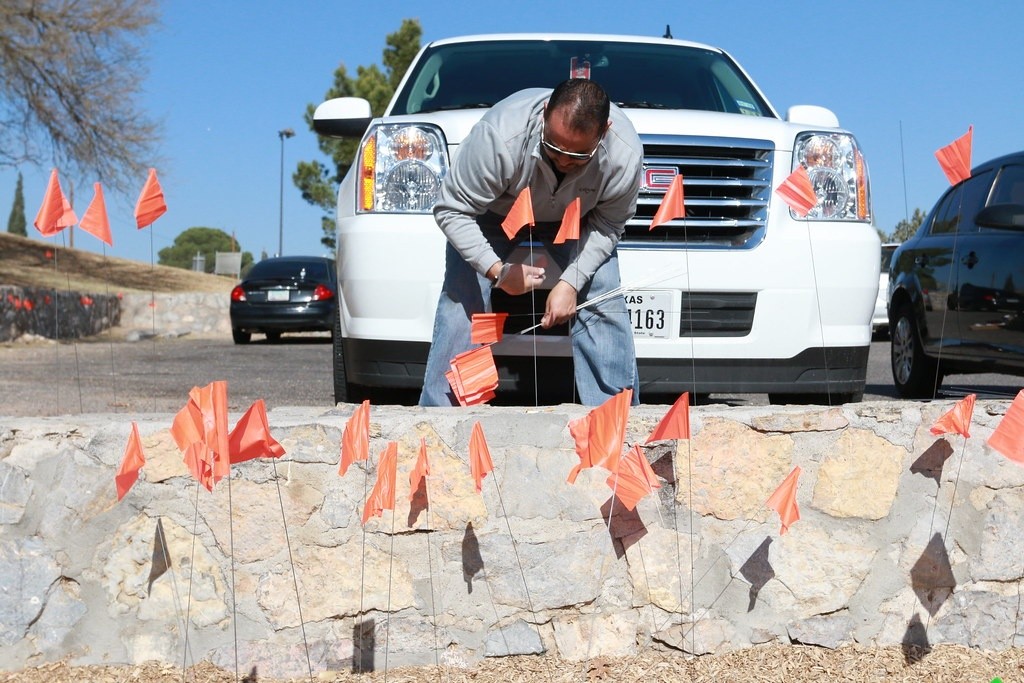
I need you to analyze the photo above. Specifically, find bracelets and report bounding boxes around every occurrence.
[489,261,509,289]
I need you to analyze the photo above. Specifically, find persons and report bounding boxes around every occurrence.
[418,78,643,407]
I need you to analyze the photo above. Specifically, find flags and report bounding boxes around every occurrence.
[650,175,685,229]
[501,185,581,245]
[929,387,1024,466]
[776,161,817,220]
[114,379,800,539]
[35,167,169,246]
[934,125,974,184]
[445,310,506,407]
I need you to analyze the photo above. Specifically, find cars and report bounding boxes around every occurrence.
[228,255,337,344]
[306,29,880,408]
[887,146,1023,403]
[868,240,908,338]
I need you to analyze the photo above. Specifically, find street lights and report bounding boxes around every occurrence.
[276,128,296,255]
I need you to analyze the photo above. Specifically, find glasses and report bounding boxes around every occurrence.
[540,117,604,161]
